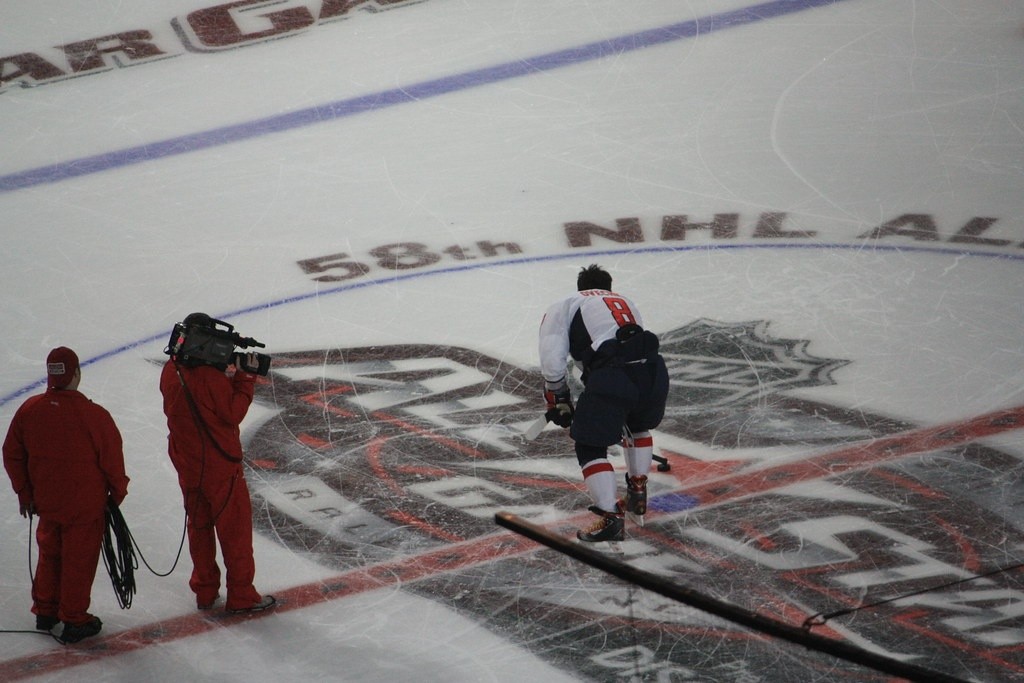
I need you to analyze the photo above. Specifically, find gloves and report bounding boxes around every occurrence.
[542,388,575,429]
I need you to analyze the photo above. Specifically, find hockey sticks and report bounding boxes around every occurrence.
[524,407,668,465]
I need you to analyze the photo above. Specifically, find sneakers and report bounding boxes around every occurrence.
[576,509,624,541]
[616,471,648,528]
[197,594,276,614]
[35,613,102,645]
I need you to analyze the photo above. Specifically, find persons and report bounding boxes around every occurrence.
[159,313,277,615]
[539,264,669,554]
[2,346,131,644]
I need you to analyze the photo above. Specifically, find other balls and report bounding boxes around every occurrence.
[657,464,670,471]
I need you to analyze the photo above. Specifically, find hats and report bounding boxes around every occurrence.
[45,345,78,388]
